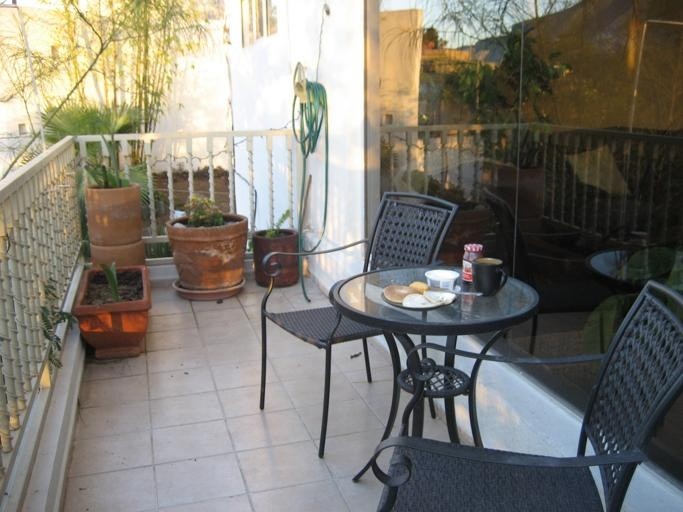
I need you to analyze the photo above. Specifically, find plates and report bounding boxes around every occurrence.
[454,285,461,294]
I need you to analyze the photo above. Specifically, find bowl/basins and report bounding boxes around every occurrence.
[425,269,459,291]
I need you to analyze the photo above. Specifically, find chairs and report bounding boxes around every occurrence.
[480,188,600,294]
[373,279,683,512]
[259,189,460,460]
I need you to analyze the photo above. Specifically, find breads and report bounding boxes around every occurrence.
[410,281,431,294]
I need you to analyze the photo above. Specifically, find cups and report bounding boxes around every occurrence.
[472,257,508,297]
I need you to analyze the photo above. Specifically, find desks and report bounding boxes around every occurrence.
[328,269,539,484]
[586,248,683,314]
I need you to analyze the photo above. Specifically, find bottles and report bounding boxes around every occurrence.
[462,244,483,285]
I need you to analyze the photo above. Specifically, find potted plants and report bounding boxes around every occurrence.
[47,98,159,268]
[74,264,149,362]
[254,207,299,289]
[167,195,247,298]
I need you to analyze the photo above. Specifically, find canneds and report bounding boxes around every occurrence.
[463,243,483,282]
